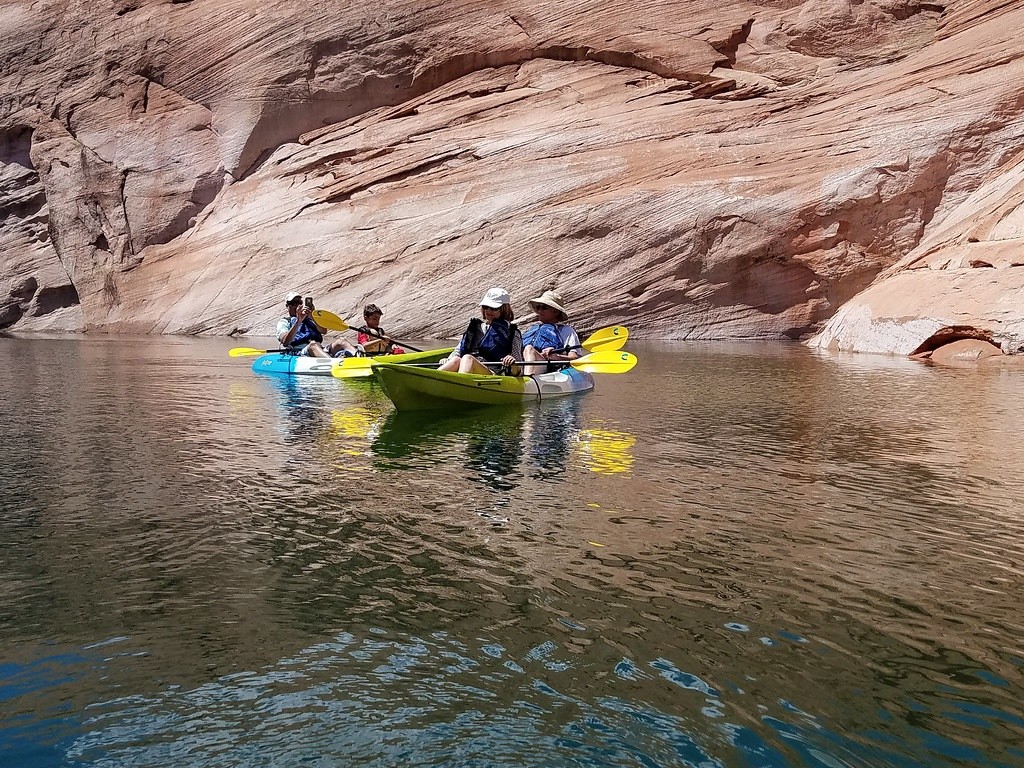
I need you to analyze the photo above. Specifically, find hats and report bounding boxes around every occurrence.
[478,287,510,309]
[285,291,302,306]
[528,291,568,321]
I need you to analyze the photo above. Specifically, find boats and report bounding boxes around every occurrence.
[251,347,457,379]
[370,361,596,412]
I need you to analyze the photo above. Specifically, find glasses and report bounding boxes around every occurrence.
[481,305,499,311]
[536,304,550,310]
[288,300,303,306]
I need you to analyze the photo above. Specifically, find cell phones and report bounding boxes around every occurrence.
[305,297,313,313]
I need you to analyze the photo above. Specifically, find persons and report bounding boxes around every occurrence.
[276,291,363,358]
[358,304,405,357]
[522,291,583,375]
[437,288,525,377]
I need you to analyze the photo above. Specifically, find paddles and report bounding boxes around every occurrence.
[541,326,628,352]
[227,346,326,357]
[313,308,427,352]
[403,349,637,374]
